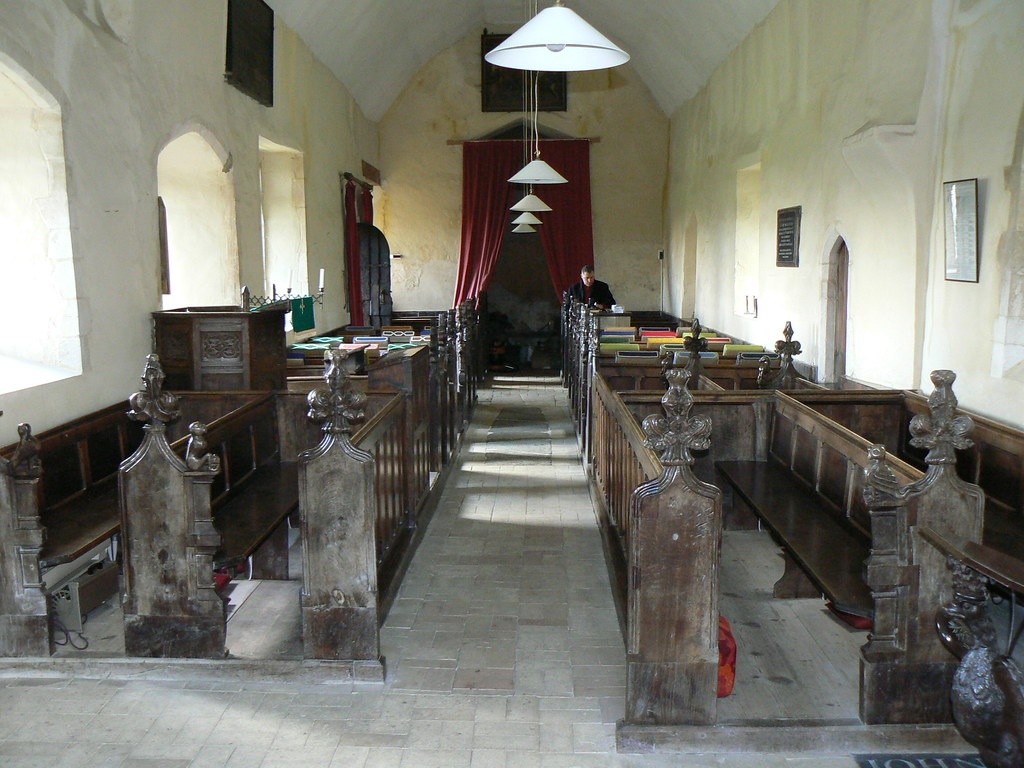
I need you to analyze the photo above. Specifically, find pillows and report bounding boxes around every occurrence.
[825,602,875,630]
[213,561,245,595]
[717,616,736,698]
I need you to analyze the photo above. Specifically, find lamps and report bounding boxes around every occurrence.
[484,0,630,72]
[508,0,568,185]
[512,1,544,225]
[511,0,539,233]
[509,2,554,212]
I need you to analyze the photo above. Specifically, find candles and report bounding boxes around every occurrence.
[320,268,325,288]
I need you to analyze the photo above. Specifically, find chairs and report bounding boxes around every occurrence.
[287,324,438,369]
[598,326,782,367]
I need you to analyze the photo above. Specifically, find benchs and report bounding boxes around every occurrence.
[169,392,300,588]
[713,388,928,617]
[0,398,131,569]
[794,377,829,389]
[698,374,726,392]
[894,394,1024,564]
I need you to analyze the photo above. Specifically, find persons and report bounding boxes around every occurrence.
[568,265,617,310]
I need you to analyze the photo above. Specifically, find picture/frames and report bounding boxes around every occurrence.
[943,178,980,283]
